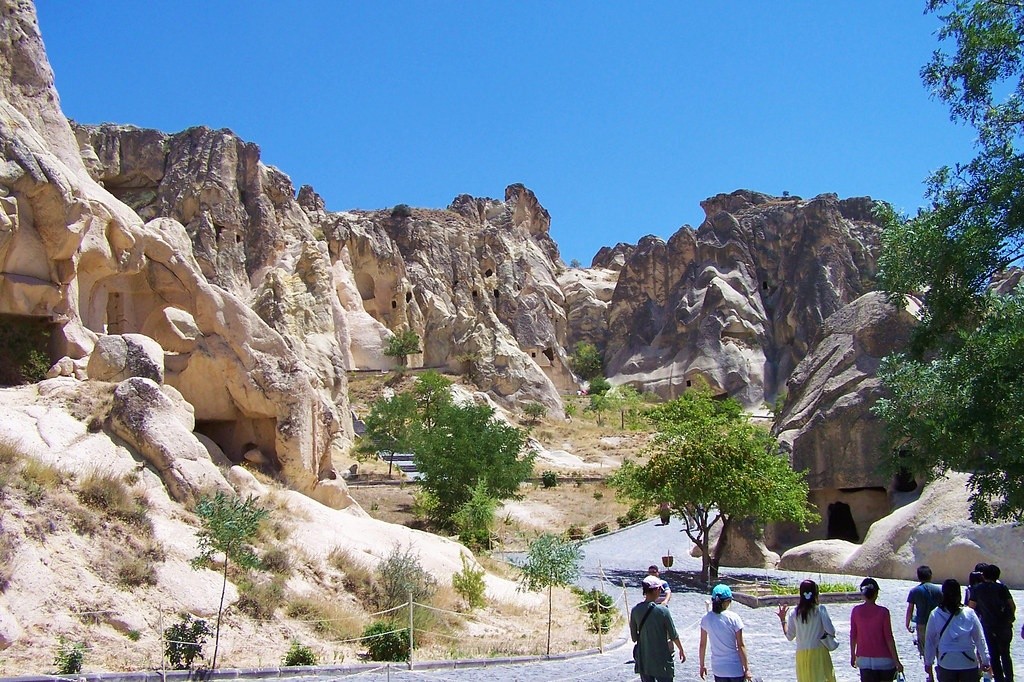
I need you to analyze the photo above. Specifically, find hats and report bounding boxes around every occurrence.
[642,575,667,589]
[712,584,734,601]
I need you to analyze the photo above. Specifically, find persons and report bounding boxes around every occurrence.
[924,579,993,682]
[649,565,675,659]
[964,563,1017,682]
[660,502,670,525]
[699,584,753,682]
[775,580,838,682]
[630,576,686,682]
[850,578,902,682]
[904,566,943,682]
[686,501,704,531]
[577,390,586,395]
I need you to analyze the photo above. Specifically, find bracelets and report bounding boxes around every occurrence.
[782,621,787,623]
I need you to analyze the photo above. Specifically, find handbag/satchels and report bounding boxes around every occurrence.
[820,632,841,652]
[633,645,640,673]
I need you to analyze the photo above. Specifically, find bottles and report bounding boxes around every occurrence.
[897,671,904,682]
[984,670,991,682]
